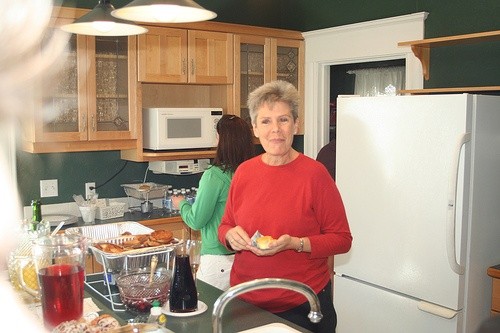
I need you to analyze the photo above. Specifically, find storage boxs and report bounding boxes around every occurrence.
[96,202,124,220]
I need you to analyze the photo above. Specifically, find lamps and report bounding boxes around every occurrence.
[61,0,149,37]
[111,0,217,24]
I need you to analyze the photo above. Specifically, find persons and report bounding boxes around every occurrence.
[218,80,353,333]
[172,113,255,291]
[316,137,336,182]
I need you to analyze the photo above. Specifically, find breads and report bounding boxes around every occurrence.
[49,314,120,333]
[256,235,276,250]
[94,228,174,253]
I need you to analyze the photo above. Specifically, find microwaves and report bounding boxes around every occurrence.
[142,107,223,150]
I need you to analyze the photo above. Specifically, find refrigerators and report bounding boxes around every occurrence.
[334,93,500,333]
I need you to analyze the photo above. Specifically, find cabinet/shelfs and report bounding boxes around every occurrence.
[233,24,306,145]
[120,22,235,161]
[23,6,137,153]
[92,221,201,273]
[397,30,500,94]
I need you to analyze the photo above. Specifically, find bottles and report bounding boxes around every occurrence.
[162,186,198,215]
[169,244,198,313]
[28,200,42,233]
[150,300,161,324]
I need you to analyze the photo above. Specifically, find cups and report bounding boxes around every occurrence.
[79,206,96,222]
[20,235,87,332]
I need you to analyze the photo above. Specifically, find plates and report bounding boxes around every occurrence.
[161,300,208,316]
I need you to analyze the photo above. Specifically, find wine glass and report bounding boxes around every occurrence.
[188,241,201,289]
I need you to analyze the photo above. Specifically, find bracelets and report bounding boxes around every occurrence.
[297,238,304,254]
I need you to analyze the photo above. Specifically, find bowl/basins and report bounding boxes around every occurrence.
[116,273,171,315]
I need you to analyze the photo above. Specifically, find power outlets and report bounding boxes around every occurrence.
[40,179,58,197]
[85,182,96,199]
